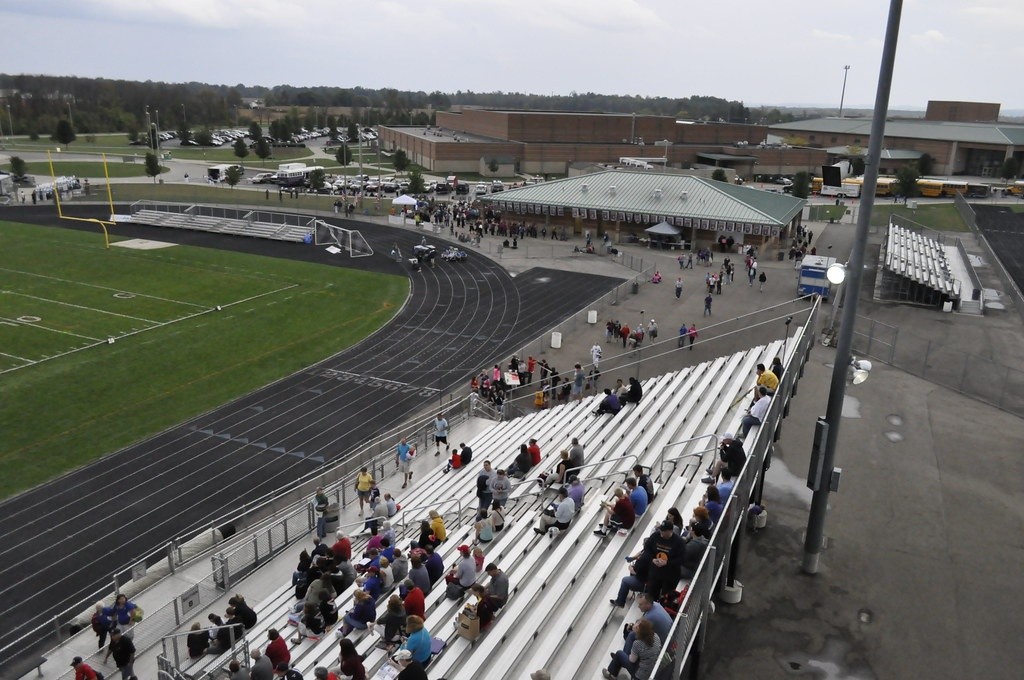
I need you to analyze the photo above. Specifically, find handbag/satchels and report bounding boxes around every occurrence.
[446,582,464,600]
[134,607,143,622]
[458,610,479,640]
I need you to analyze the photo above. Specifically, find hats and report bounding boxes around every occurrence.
[720,433,733,439]
[70,656,82,666]
[531,669,551,680]
[458,545,470,553]
[657,521,673,531]
[394,650,412,661]
[365,565,378,572]
[692,521,705,535]
[110,628,120,635]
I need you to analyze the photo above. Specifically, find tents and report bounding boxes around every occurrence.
[392,195,418,207]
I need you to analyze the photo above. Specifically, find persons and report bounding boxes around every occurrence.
[644,232,677,251]
[787,224,816,267]
[703,293,712,317]
[20,175,91,205]
[705,257,735,297]
[204,173,221,185]
[678,246,714,270]
[391,194,612,273]
[606,319,658,351]
[184,172,189,183]
[593,357,784,680]
[265,186,384,219]
[651,270,662,283]
[678,323,688,348]
[688,323,699,350]
[675,277,683,300]
[66,412,586,679]
[470,339,604,409]
[742,239,767,294]
[717,235,735,253]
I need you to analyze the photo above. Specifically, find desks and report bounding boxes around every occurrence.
[639,239,691,250]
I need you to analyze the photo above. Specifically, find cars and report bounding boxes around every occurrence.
[159,126,547,196]
[783,183,794,194]
[774,176,792,185]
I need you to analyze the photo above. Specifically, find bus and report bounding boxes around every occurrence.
[809,176,1024,199]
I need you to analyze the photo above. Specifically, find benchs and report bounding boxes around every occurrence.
[178,326,805,680]
[130,208,314,242]
[887,222,960,295]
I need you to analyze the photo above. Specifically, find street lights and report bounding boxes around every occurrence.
[314,106,320,128]
[838,65,851,119]
[355,123,364,207]
[151,122,160,151]
[6,104,14,145]
[156,109,159,131]
[146,105,149,133]
[408,111,415,127]
[145,112,151,139]
[67,102,73,131]
[182,103,186,125]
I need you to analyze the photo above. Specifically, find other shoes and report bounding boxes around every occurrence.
[402,483,407,488]
[446,445,449,449]
[609,599,625,609]
[594,530,606,537]
[435,452,440,456]
[706,468,713,474]
[409,472,413,479]
[291,638,300,644]
[359,509,364,516]
[534,528,545,536]
[599,523,612,528]
[701,476,715,484]
[602,668,617,680]
